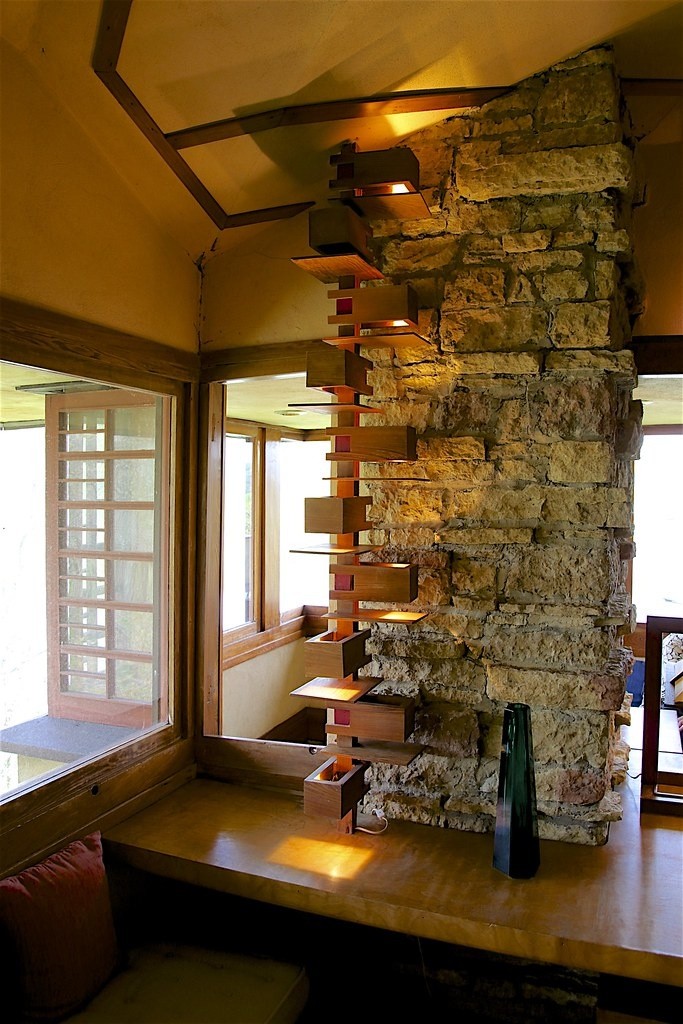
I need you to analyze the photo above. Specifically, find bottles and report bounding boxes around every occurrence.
[492,702,541,880]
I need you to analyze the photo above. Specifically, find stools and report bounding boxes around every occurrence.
[75,943,310,1024]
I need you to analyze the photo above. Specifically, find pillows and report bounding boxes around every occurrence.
[0,832,120,1023]
